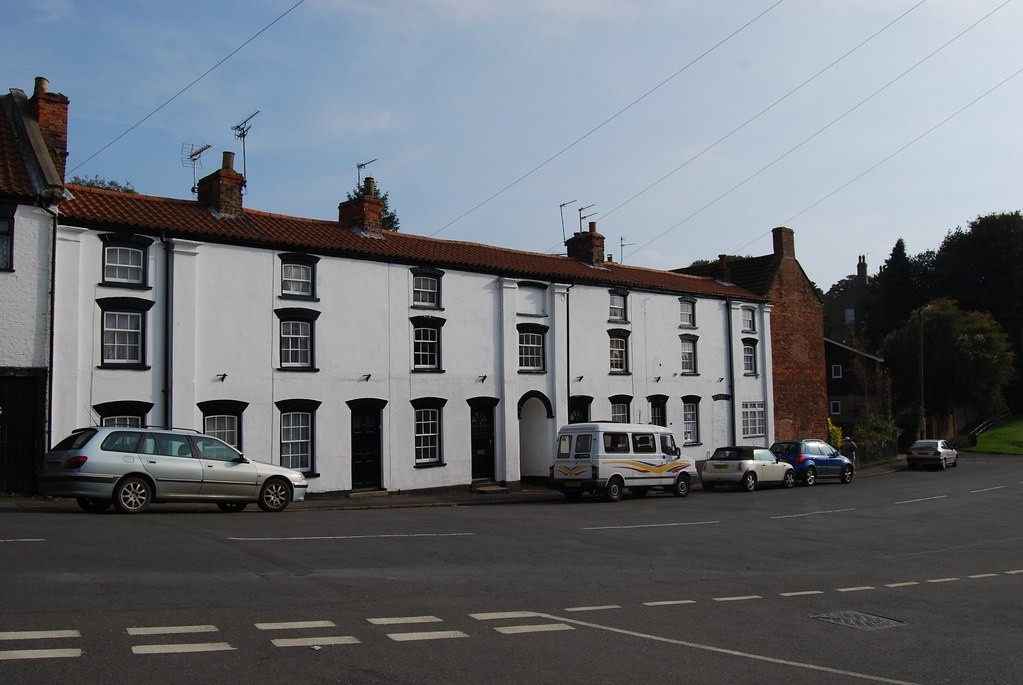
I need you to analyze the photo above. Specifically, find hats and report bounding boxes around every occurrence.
[845,437,850,440]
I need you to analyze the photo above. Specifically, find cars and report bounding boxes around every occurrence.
[907,438,959,470]
[702,446,796,492]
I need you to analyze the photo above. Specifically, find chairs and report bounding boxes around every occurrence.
[638,437,653,453]
[178,444,191,456]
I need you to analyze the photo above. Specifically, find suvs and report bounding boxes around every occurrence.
[770,438,856,487]
[30,425,308,515]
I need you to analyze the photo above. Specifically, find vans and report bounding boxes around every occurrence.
[549,421,700,503]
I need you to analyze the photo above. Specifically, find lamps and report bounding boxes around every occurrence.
[478,375,487,383]
[363,374,372,382]
[577,376,583,382]
[217,373,228,382]
[719,378,724,382]
[654,376,661,383]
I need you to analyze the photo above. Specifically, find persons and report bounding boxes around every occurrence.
[840,437,857,475]
